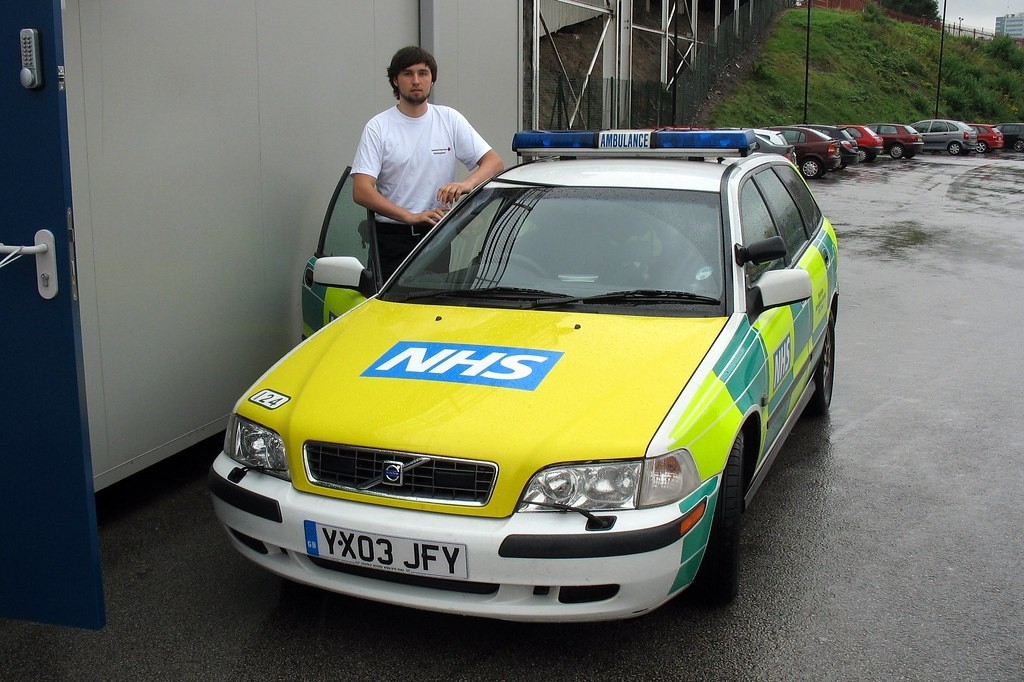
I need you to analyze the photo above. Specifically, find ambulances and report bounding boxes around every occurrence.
[212,129,836,623]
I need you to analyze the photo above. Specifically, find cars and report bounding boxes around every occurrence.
[995,123,1024,151]
[908,120,1003,156]
[740,124,886,179]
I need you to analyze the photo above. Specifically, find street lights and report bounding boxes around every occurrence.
[959,17,964,38]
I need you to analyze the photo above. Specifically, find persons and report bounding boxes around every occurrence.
[350,47,505,281]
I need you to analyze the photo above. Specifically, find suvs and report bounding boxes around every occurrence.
[864,123,925,161]
